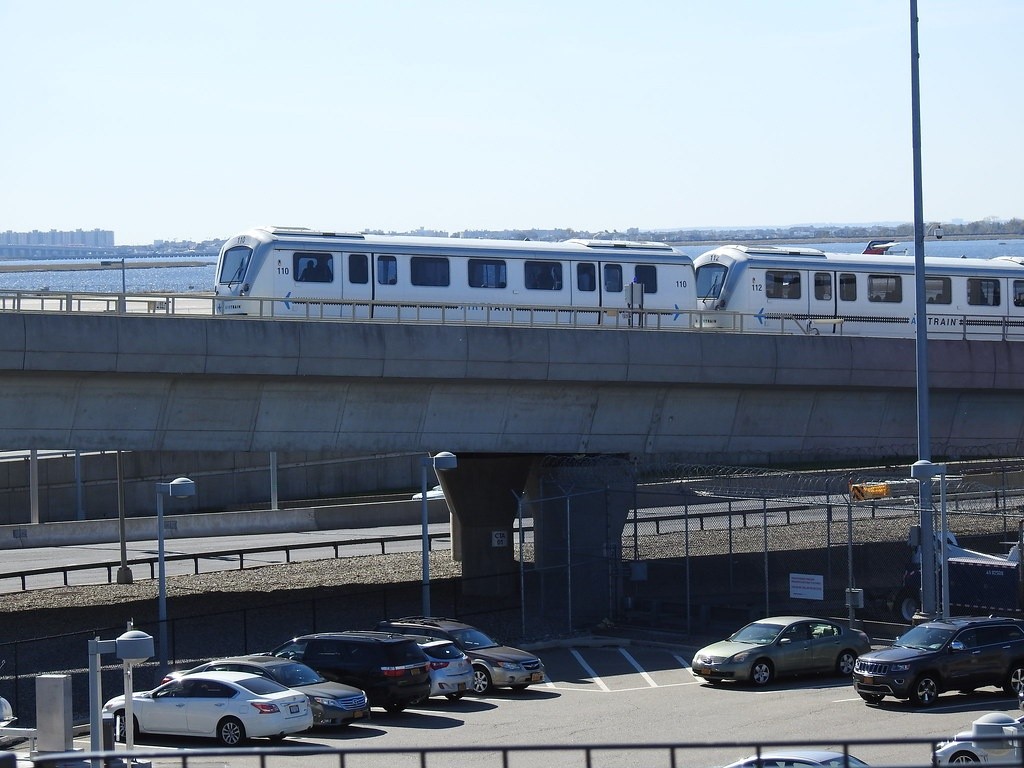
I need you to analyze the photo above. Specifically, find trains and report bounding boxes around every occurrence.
[212,226,1024,343]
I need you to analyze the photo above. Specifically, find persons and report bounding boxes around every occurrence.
[300,260,331,282]
[872,290,1024,306]
[788,277,826,300]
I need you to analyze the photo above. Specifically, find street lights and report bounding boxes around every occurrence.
[156,477,196,684]
[99,256,125,293]
[911,460,950,620]
[422,449,458,618]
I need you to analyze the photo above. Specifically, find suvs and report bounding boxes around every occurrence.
[262,630,432,715]
[373,616,547,696]
[852,616,1024,709]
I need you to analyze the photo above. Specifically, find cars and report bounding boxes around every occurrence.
[931,734,988,765]
[692,617,871,688]
[402,634,474,708]
[160,654,370,734]
[723,748,870,768]
[103,671,313,747]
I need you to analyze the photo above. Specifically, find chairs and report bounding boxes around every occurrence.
[796,624,810,640]
